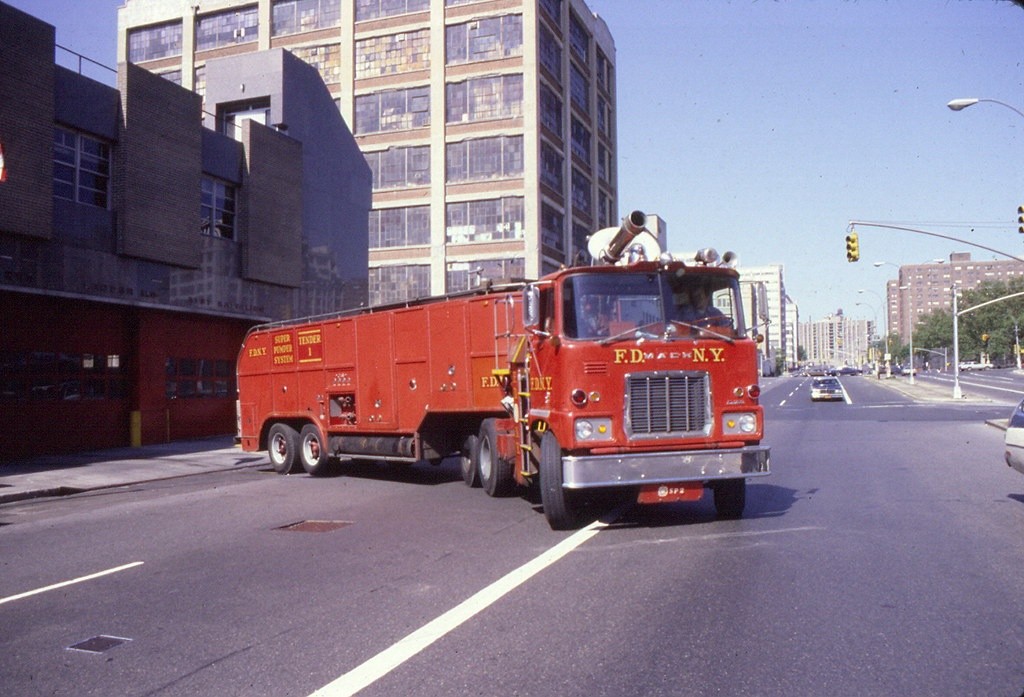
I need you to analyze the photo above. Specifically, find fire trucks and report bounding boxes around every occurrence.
[231,209,777,532]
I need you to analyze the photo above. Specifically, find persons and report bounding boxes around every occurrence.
[676,287,728,336]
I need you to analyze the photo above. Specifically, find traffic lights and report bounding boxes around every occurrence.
[845,233,860,263]
[1016,207,1024,233]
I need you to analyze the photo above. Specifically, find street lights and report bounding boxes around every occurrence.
[856,300,891,375]
[857,287,910,379]
[873,257,945,383]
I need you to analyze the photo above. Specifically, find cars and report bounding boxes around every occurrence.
[959,360,996,371]
[792,365,901,377]
[810,377,844,401]
[901,365,918,376]
[1003,393,1024,478]
